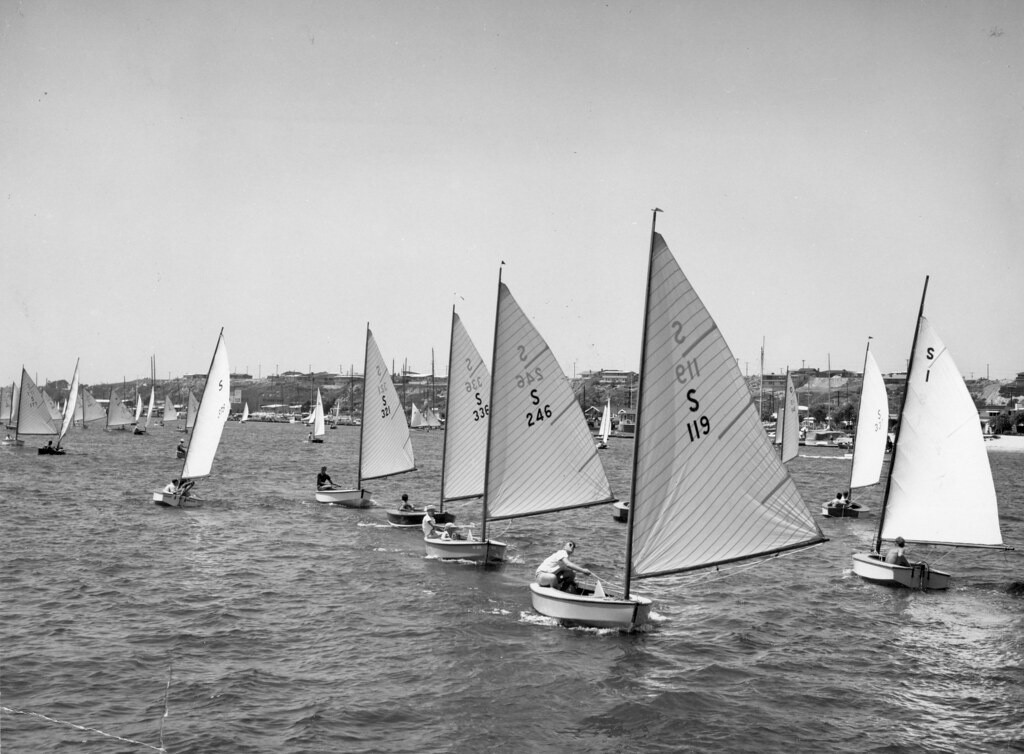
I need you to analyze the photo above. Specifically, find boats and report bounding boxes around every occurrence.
[805,429,853,447]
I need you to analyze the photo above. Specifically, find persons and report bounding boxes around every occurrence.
[535,541,591,593]
[163,479,179,494]
[177,439,186,457]
[308,432,313,440]
[421,506,457,541]
[399,494,414,512]
[48,441,54,450]
[316,466,340,490]
[884,537,910,567]
[6,434,13,440]
[828,492,849,508]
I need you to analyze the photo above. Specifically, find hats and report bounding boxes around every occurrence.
[179,438,184,442]
[424,505,437,512]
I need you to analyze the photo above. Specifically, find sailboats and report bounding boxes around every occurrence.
[822,274,1014,592]
[71,386,107,429]
[38,358,79,455]
[315,321,418,508]
[423,261,620,566]
[530,207,831,631]
[330,402,340,429]
[158,395,177,426]
[410,402,441,433]
[0,362,63,446]
[104,386,133,432]
[241,401,249,423]
[133,386,155,435]
[153,327,231,507]
[309,387,325,443]
[595,397,612,449]
[176,388,201,433]
[774,366,800,463]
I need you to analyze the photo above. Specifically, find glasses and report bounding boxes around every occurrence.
[569,543,574,550]
